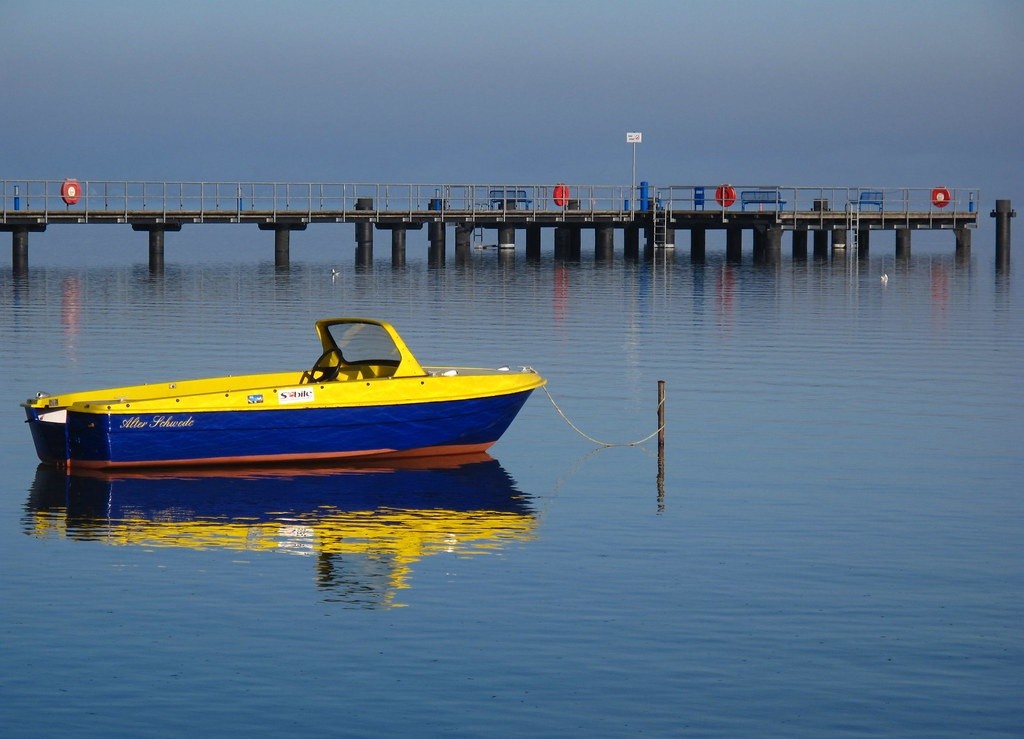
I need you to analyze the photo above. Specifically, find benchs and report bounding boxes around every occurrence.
[488,190,532,210]
[844,192,884,212]
[741,191,787,212]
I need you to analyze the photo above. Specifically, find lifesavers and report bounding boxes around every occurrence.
[932,186,951,207]
[716,184,735,206]
[62,182,81,204]
[553,183,569,205]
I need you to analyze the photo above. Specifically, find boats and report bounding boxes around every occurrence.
[20,318,548,470]
